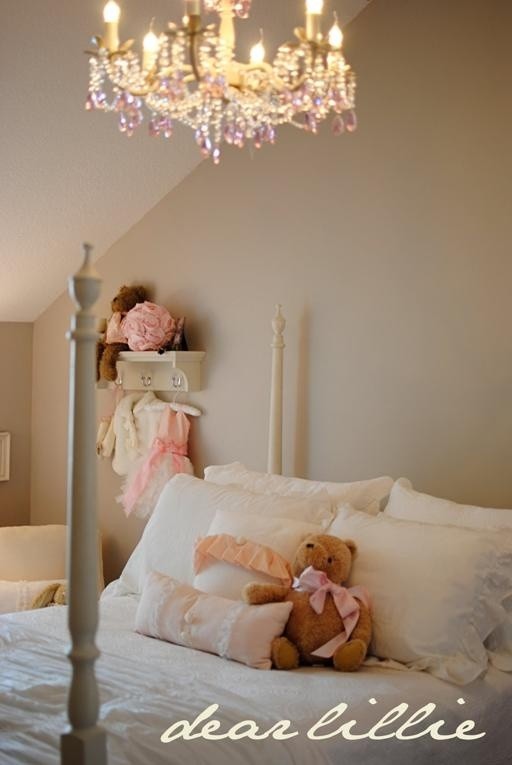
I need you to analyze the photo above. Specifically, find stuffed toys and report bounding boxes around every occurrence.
[242,531,372,673]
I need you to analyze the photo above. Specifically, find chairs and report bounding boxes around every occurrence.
[0,523,105,616]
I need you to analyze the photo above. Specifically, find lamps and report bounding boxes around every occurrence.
[81,1,359,166]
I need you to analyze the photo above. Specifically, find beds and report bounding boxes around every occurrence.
[0,241,512,765]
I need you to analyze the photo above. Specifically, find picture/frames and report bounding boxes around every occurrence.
[0,430,10,481]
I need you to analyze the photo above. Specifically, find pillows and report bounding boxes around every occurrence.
[0,580,66,613]
